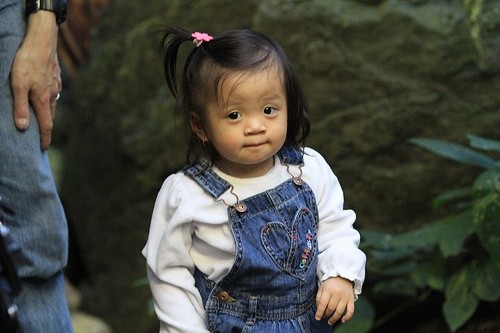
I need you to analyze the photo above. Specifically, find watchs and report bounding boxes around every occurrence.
[23,0,67,25]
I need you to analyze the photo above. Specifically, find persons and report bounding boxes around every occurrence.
[141,25,367,333]
[0,0,74,333]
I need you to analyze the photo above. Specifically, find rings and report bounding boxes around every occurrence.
[55,93,60,101]
